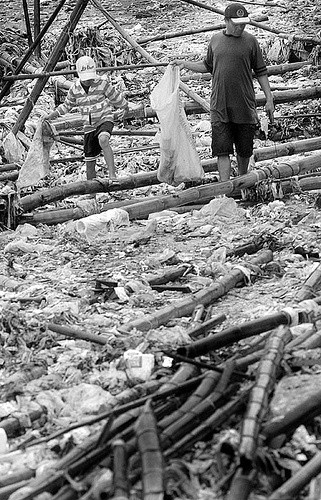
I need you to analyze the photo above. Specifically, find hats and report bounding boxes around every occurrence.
[223,4,251,24]
[76,55,100,81]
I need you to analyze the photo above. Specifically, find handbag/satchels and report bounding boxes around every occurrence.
[148,60,207,184]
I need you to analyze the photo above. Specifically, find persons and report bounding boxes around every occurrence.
[167,4,277,204]
[40,54,137,187]
